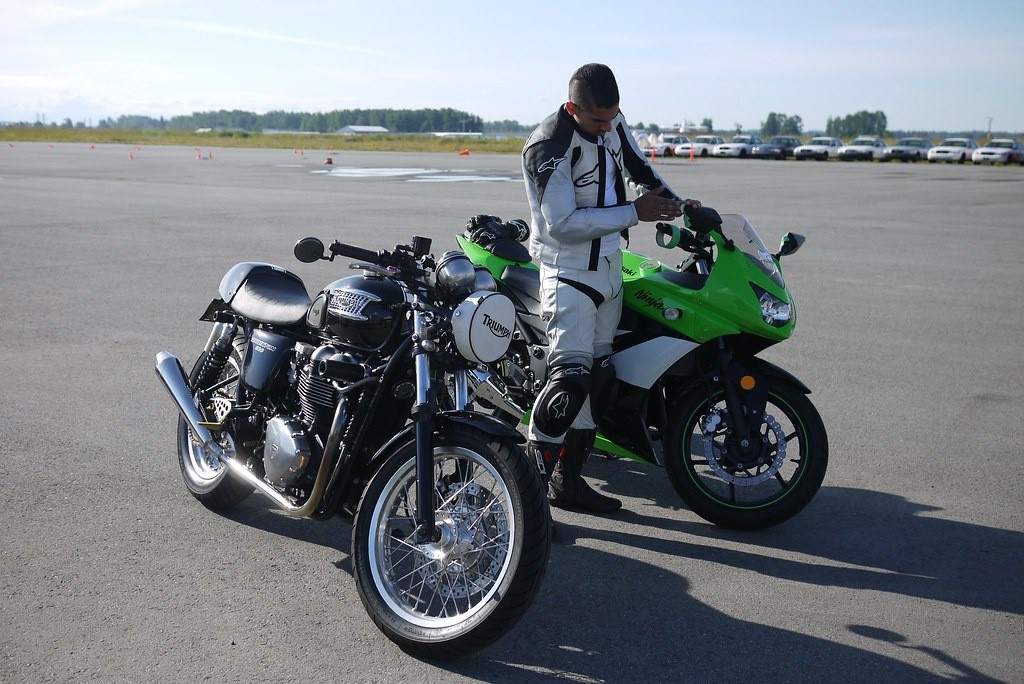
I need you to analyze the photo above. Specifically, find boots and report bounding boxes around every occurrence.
[528,440,563,544]
[548,427,622,512]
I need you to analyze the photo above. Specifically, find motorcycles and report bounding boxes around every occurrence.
[450,178,831,530]
[153,234,554,660]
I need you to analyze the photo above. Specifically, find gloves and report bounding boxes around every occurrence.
[470,218,529,248]
[467,214,502,234]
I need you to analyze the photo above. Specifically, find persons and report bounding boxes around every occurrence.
[522,62,705,539]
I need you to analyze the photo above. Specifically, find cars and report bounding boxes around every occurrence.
[838,134,890,163]
[675,135,727,157]
[971,136,1024,166]
[884,137,934,163]
[712,134,764,158]
[927,137,981,164]
[752,136,804,160]
[793,137,846,162]
[638,134,691,157]
